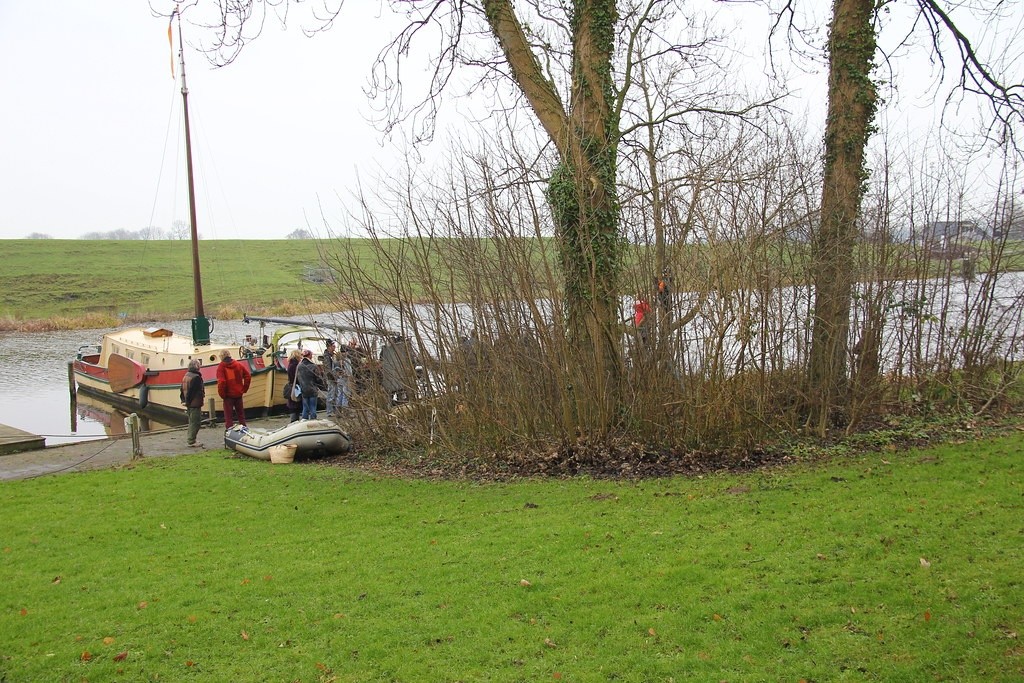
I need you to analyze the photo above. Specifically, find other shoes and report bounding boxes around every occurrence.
[188,442,203,447]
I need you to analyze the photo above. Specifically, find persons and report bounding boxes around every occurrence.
[450,331,494,378]
[332,344,353,412]
[348,337,417,406]
[652,268,674,322]
[179,359,204,446]
[631,293,651,348]
[285,349,303,423]
[215,348,251,428]
[297,349,326,419]
[323,338,337,417]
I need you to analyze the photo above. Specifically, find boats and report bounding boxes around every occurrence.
[224,416,351,463]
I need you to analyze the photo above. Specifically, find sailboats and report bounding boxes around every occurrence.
[74,2,330,427]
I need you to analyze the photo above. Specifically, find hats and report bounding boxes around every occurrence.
[326,339,334,347]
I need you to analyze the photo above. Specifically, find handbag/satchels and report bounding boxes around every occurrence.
[291,387,301,402]
[283,383,291,399]
